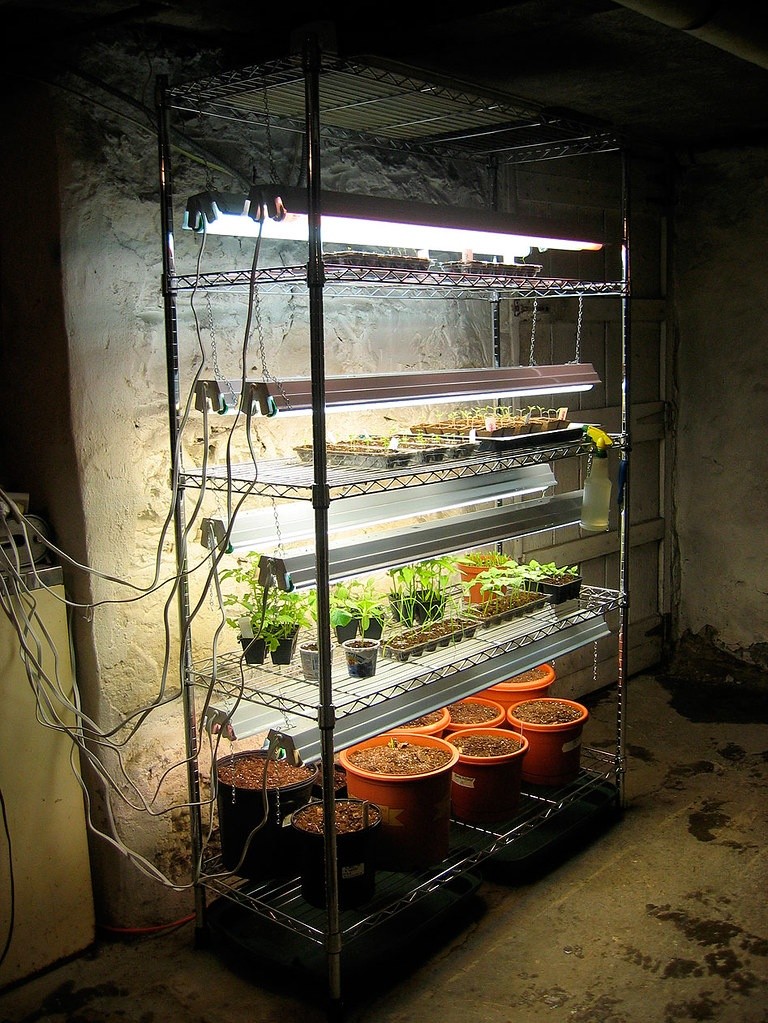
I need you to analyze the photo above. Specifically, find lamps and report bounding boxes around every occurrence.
[197,464,598,591]
[188,76,608,255]
[190,294,601,417]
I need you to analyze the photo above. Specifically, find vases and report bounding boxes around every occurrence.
[212,665,590,907]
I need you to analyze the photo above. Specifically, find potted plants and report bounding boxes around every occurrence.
[217,547,585,681]
[292,403,603,467]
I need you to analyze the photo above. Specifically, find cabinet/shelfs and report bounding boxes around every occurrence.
[154,29,631,1023]
